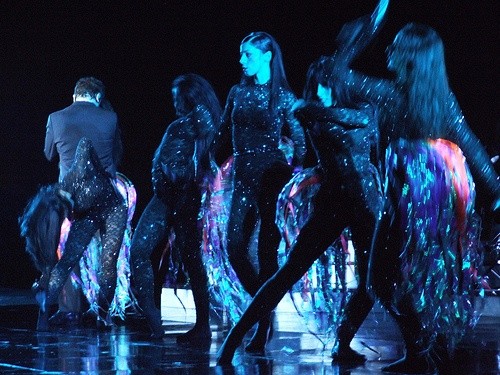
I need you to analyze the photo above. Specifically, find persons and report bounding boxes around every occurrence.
[130,74,223,343]
[217,58,387,369]
[219,32,306,354]
[320,0,500,375]
[40,77,127,330]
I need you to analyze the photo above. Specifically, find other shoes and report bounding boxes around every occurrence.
[176,328,212,344]
[48,310,80,328]
[36,303,49,331]
[246,334,266,353]
[216,333,242,365]
[380,353,431,374]
[96,317,111,331]
[335,346,367,364]
